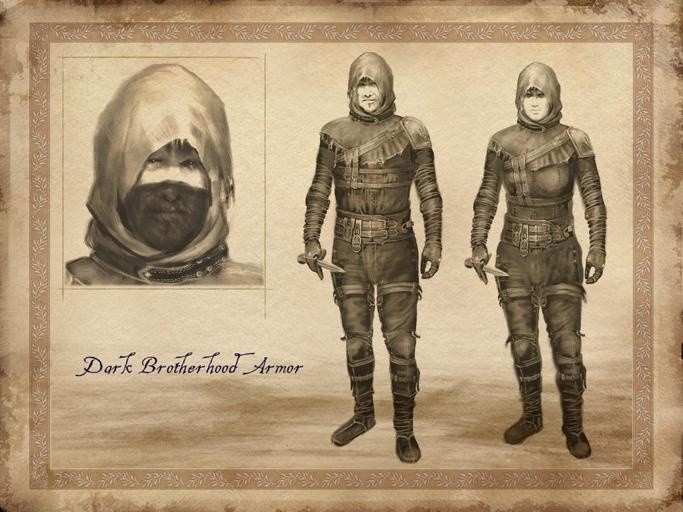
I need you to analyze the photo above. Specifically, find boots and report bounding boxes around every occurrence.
[388,357,421,464]
[331,356,376,447]
[503,357,544,445]
[555,356,590,459]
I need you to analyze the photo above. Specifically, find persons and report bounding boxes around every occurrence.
[65,62,265,288]
[464,61,608,458]
[297,50,443,464]
